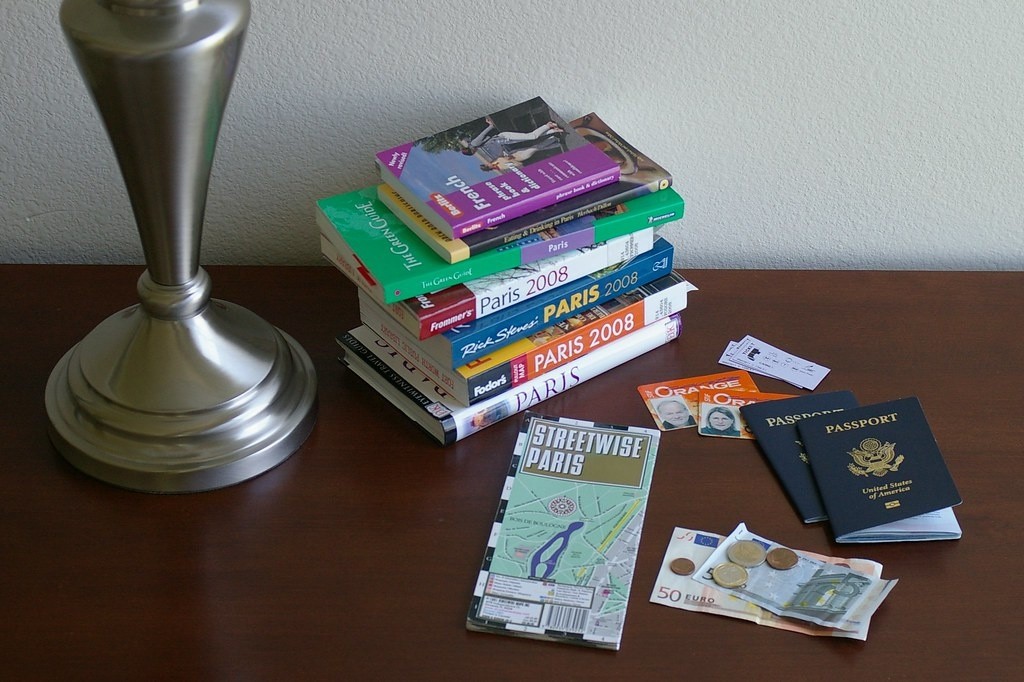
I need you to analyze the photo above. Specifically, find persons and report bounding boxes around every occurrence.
[458,119,563,156]
[656,401,697,429]
[479,135,562,172]
[700,406,741,437]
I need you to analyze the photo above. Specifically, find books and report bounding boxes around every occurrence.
[310,96,698,445]
[464,409,660,651]
